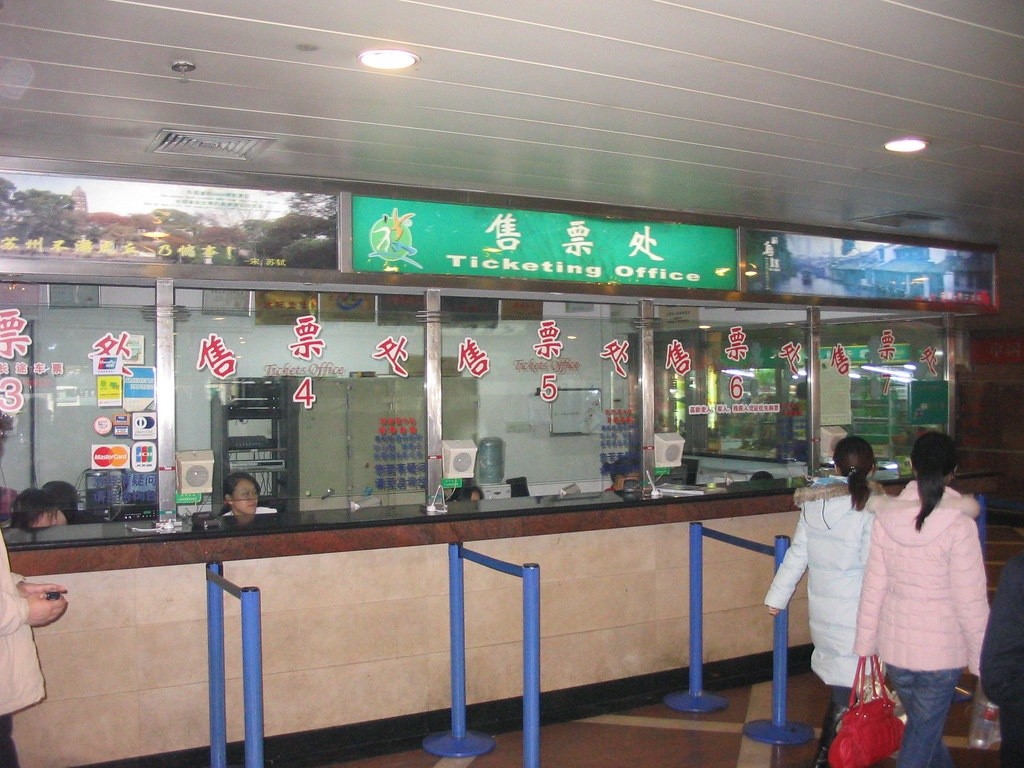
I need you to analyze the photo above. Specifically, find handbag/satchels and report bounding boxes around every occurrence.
[828,653,908,768]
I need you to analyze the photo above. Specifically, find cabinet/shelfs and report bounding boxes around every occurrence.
[294,374,481,512]
[210,376,300,514]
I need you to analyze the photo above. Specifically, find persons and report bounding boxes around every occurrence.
[783,382,809,462]
[750,471,774,481]
[219,472,277,516]
[459,486,485,500]
[764,431,1024,768]
[602,454,643,492]
[0,410,78,768]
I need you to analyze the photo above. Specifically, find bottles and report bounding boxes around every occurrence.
[971,700,998,748]
[480,439,504,484]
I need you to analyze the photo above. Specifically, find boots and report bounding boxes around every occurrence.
[814,703,848,768]
